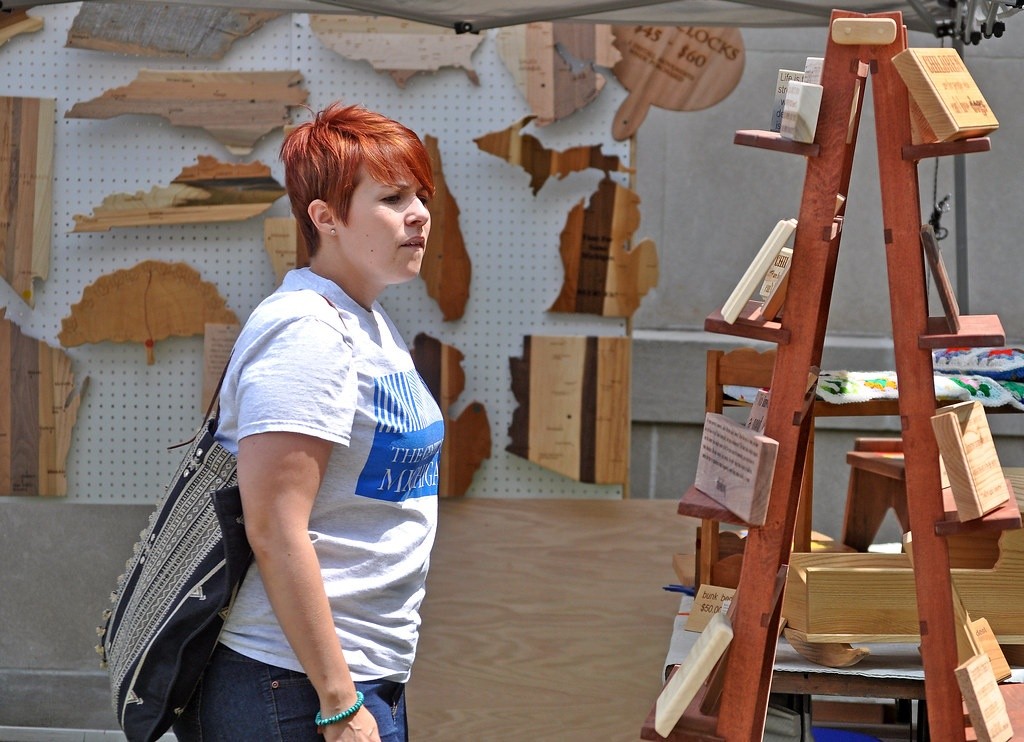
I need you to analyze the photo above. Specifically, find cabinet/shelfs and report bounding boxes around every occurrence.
[639,8,1024,742]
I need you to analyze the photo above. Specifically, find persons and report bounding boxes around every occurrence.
[169,104,444,742]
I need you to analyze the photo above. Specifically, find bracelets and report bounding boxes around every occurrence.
[315,691,364,734]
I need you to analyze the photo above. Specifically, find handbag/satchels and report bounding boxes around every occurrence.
[94,289,346,742]
